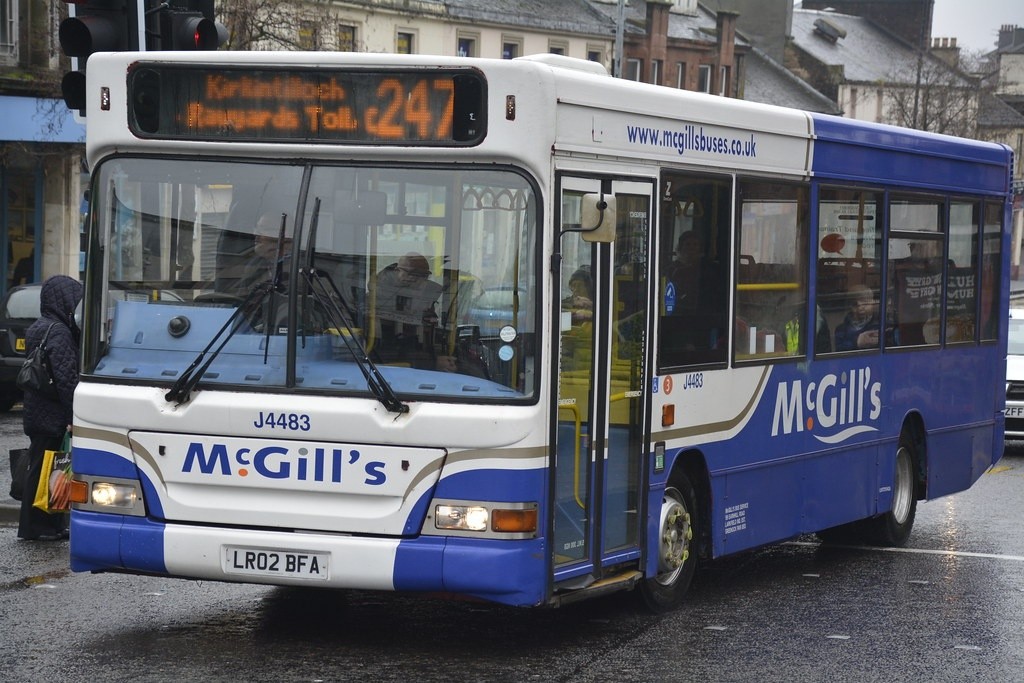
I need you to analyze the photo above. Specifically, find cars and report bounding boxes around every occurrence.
[0,281,185,415]
[1006,308,1024,444]
[462,283,527,339]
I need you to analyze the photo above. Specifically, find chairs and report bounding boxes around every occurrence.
[741,255,774,304]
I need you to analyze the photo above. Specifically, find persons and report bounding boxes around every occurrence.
[18,273,84,540]
[213,166,492,379]
[562,230,899,354]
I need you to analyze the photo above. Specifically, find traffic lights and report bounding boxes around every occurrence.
[58,0,140,118]
[161,8,220,51]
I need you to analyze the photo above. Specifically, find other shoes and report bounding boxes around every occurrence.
[25,528,71,541]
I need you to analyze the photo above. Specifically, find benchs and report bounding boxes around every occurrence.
[818,256,894,291]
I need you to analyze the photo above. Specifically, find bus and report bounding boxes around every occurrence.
[66,51,1016,614]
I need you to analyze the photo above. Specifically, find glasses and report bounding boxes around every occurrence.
[399,268,431,281]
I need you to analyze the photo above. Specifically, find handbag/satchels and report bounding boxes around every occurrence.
[9,441,33,501]
[33,429,72,514]
[17,321,61,399]
